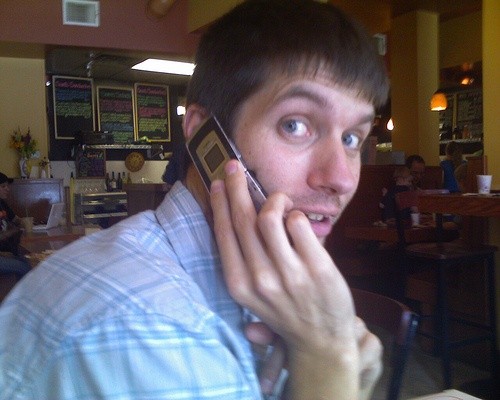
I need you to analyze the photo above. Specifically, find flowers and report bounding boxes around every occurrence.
[9,125,40,157]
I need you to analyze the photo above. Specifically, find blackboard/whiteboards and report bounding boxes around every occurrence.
[51,74,183,161]
[53,76,99,138]
[74,146,106,180]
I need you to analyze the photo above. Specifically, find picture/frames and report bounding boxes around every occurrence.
[439,91,455,141]
[454,89,482,143]
[51,73,96,141]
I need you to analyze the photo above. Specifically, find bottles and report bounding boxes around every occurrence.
[127,173,133,184]
[462,125,470,141]
[121,171,127,184]
[453,125,460,140]
[110,171,117,192]
[117,172,123,190]
[105,173,110,192]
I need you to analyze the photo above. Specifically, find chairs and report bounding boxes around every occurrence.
[393,189,499,356]
[351,286,420,399]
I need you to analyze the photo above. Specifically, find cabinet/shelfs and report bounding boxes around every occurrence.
[65,177,130,228]
[4,178,66,229]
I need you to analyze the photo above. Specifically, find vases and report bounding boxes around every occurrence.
[18,154,32,179]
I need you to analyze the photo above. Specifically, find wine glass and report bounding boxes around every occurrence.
[79,183,103,193]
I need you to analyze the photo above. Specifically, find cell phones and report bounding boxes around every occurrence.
[186,113,280,236]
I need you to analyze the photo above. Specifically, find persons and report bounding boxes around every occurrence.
[406,155,426,190]
[0,172,32,283]
[443,142,474,238]
[0,1,391,400]
[379,166,413,224]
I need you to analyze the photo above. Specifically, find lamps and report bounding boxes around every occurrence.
[429,76,481,112]
[175,95,189,117]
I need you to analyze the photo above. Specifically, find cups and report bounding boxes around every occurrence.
[476,174,493,196]
[411,213,421,227]
[23,217,33,233]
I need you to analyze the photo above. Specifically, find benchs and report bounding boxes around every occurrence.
[325,163,446,281]
